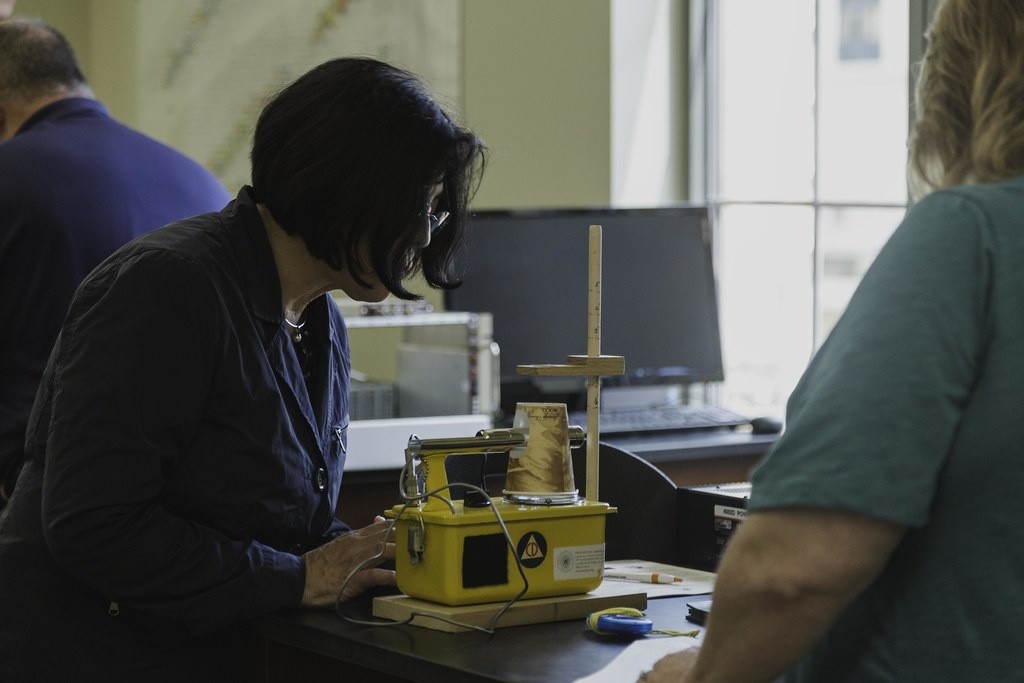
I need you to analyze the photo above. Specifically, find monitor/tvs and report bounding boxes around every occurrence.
[444,206,725,414]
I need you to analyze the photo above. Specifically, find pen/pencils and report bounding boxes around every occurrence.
[602,570,684,585]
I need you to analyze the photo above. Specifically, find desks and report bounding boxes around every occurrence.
[599,423,787,487]
[241,589,713,683]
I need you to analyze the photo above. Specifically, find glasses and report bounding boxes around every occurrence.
[414,202,451,238]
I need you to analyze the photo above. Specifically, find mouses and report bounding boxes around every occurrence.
[752,418,783,435]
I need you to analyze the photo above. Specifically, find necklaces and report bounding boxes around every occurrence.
[284,318,306,342]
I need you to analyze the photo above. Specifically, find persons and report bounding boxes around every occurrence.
[0,56,488,683]
[0,23,233,500]
[641,2,1024,680]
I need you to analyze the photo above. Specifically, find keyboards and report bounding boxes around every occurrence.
[568,409,751,436]
